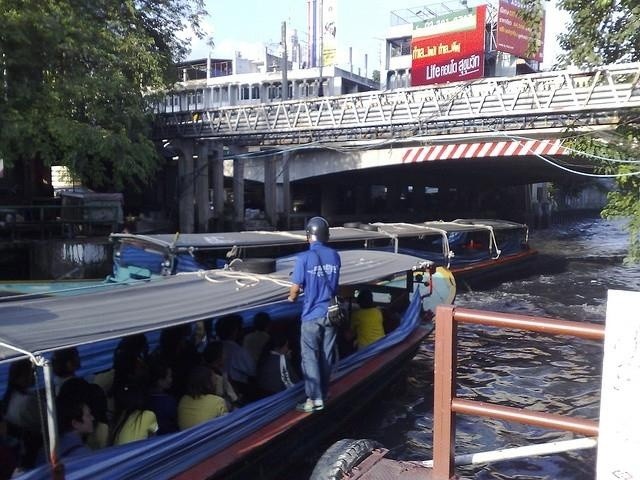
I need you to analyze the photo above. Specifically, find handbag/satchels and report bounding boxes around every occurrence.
[324,295,347,327]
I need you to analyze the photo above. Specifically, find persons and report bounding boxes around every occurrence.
[345,286,385,352]
[288,215,341,413]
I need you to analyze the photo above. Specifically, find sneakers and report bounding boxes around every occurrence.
[295,398,324,413]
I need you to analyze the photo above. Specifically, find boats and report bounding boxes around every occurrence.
[109,217,538,280]
[1,248,458,478]
[1,186,126,238]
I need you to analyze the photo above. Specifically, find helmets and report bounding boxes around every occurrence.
[305,217,331,242]
